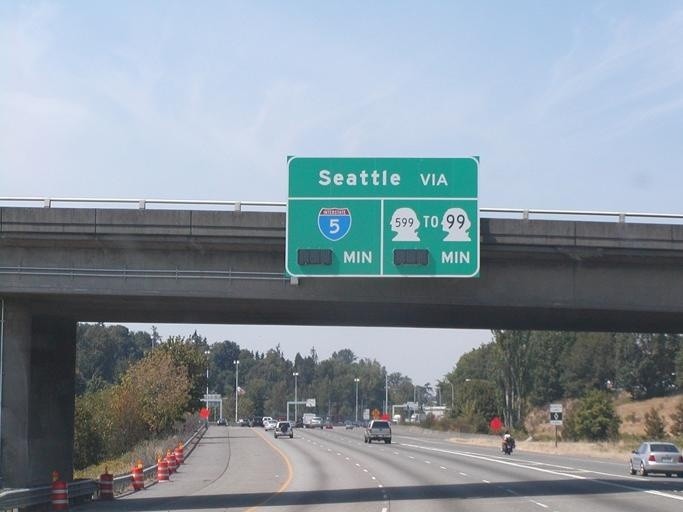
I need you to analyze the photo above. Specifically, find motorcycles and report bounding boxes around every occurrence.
[502,437,513,455]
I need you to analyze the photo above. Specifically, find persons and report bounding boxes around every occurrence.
[504,430,510,440]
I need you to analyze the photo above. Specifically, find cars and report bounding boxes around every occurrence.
[303,413,353,430]
[629,442,683,478]
[217,416,292,439]
[364,420,391,444]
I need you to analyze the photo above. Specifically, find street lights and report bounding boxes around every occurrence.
[205,349,210,423]
[385,375,391,414]
[234,360,240,422]
[354,377,360,423]
[292,371,299,422]
